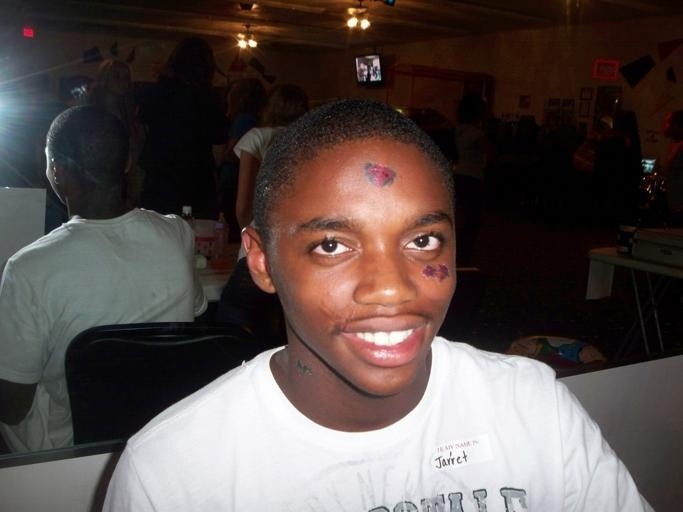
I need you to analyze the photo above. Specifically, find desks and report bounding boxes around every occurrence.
[587,244,683,361]
[193,259,480,303]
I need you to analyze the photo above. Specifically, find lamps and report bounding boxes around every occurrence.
[235,22,259,50]
[345,7,372,31]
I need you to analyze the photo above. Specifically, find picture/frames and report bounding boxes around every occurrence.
[591,57,621,81]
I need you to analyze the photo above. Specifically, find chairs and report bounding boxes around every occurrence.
[59,322,253,448]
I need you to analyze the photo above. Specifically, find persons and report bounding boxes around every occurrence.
[101,98,653,512]
[58,37,146,209]
[450,101,496,184]
[137,36,226,220]
[0,105,209,454]
[656,109,683,211]
[217,76,308,356]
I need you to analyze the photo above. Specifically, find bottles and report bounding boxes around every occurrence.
[181,206,193,222]
[215,211,228,251]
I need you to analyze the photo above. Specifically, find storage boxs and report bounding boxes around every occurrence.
[631,228,683,267]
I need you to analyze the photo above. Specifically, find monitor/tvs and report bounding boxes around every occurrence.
[355,53,383,84]
